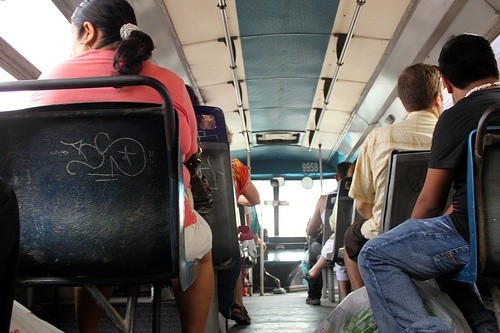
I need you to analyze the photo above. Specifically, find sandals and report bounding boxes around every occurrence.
[231,303,251,325]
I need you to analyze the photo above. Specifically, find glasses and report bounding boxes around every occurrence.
[432,65,442,99]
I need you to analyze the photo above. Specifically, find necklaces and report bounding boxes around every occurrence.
[465,81,500,97]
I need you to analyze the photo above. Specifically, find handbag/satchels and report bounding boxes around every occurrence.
[184,146,213,216]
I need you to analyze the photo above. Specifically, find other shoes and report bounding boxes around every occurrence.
[306,297,320,305]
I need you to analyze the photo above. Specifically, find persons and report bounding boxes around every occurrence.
[358,34,500,332]
[305,162,352,305]
[245,206,267,250]
[272,179,279,186]
[31,0,214,333]
[342,63,445,293]
[201,114,261,325]
[303,159,357,300]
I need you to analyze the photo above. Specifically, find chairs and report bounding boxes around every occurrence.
[0,76,241,333]
[317,102,500,333]
